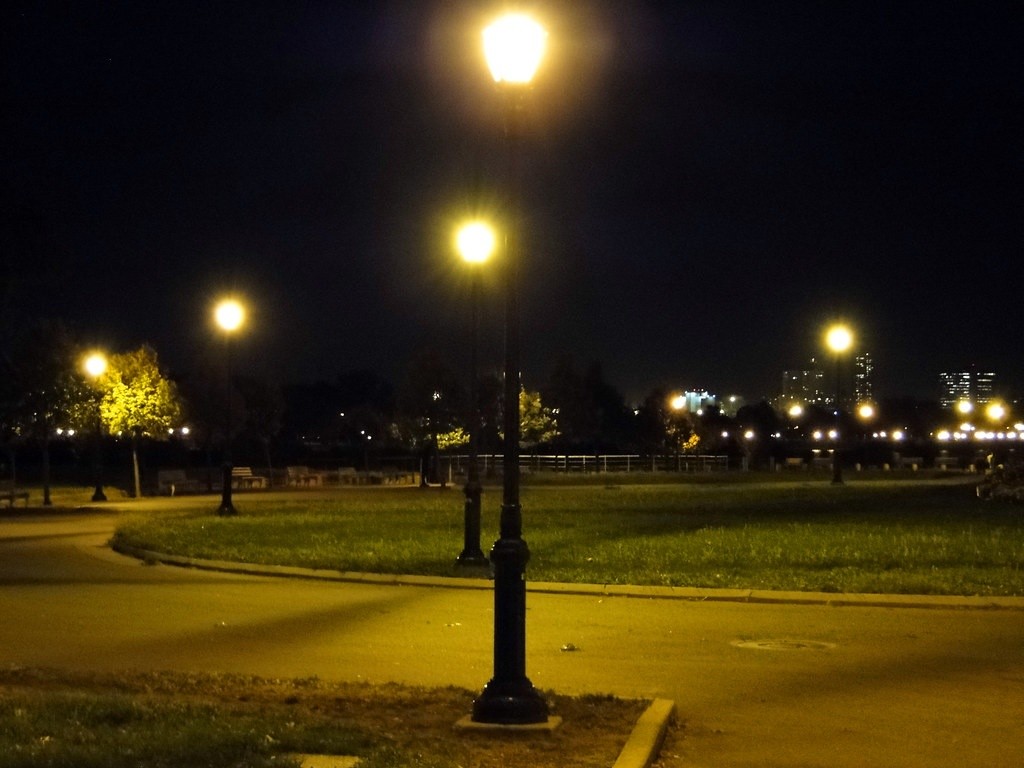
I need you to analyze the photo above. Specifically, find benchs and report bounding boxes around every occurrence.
[0,479,30,508]
[231,466,266,489]
[286,465,320,488]
[338,466,372,485]
[381,465,408,484]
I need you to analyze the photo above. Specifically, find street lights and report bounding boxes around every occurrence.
[822,323,854,484]
[454,218,494,567]
[216,299,245,516]
[81,352,110,501]
[470,9,551,724]
